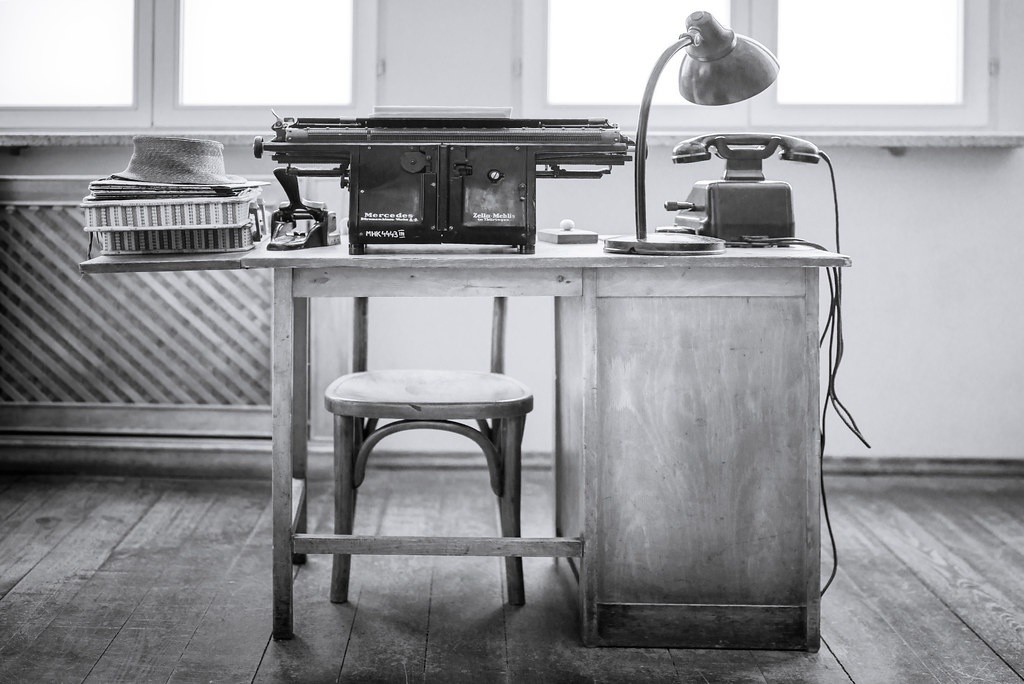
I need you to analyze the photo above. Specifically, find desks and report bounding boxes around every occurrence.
[78,232,851,653]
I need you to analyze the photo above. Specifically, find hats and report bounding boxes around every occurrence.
[111,135,247,185]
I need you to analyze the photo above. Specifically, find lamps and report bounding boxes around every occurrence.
[600,11,780,258]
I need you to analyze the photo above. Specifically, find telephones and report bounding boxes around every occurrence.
[664,130,822,248]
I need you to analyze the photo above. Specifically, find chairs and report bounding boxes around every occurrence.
[322,296,535,608]
